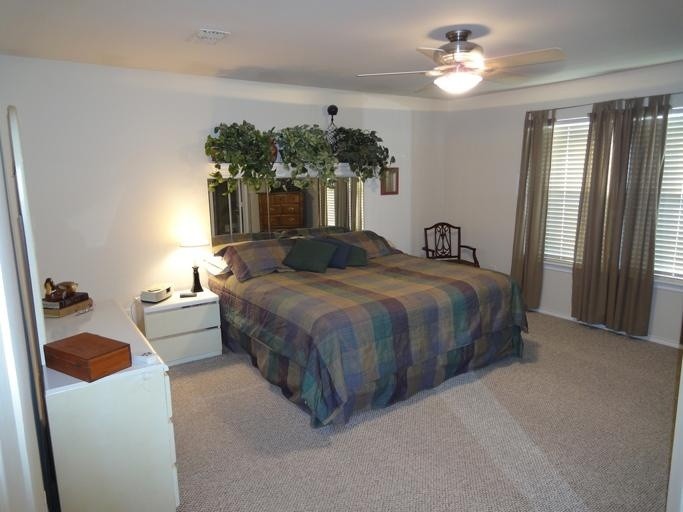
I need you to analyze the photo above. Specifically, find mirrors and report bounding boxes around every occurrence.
[206,178,363,239]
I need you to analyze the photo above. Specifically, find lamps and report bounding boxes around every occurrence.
[171,241,213,292]
[431,67,484,94]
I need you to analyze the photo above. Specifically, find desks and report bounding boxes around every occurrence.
[41,273,183,512]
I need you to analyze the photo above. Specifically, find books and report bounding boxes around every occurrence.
[42,292,88,309]
[43,297,93,320]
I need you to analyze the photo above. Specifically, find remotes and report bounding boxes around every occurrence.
[180,293,196,297]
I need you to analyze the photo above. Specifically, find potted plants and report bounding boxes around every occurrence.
[329,124,393,186]
[205,123,289,200]
[277,124,340,188]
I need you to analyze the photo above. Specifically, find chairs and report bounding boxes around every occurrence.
[421,222,481,268]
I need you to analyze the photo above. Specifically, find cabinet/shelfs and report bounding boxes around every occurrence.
[256,190,304,230]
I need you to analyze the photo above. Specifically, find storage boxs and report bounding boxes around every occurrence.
[43,333,131,382]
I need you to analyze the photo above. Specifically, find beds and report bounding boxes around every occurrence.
[207,229,529,430]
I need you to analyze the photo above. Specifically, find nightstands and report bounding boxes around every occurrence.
[134,290,222,366]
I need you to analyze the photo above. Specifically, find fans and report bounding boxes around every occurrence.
[356,28,561,95]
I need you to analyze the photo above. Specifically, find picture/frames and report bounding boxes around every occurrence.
[380,168,400,194]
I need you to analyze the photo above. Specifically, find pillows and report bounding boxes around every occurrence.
[208,231,283,246]
[215,239,297,283]
[280,226,351,236]
[347,244,368,267]
[313,236,352,271]
[282,238,337,272]
[329,231,403,259]
[203,241,255,276]
